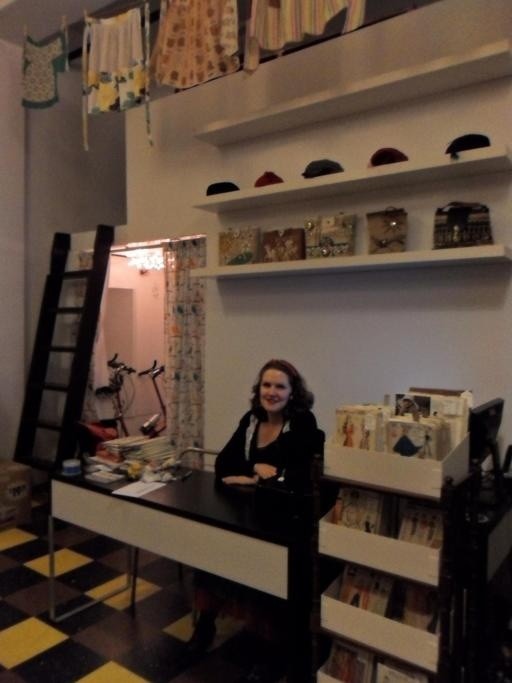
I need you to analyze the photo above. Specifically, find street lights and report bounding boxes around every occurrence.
[216,194,495,264]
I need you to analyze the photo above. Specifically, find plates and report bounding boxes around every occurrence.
[318,383,512,683]
[190,37,512,283]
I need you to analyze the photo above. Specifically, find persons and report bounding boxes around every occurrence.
[176,355,326,664]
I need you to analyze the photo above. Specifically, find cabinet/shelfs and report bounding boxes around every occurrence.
[143,412,162,431]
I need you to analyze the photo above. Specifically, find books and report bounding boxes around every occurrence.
[338,565,440,631]
[334,487,442,549]
[326,386,476,461]
[85,433,195,484]
[328,640,428,681]
[0,460,32,530]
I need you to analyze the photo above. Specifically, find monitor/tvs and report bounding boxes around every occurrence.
[85,433,195,484]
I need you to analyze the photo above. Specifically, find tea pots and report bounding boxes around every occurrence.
[106,286,135,405]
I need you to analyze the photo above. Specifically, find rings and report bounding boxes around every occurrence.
[181,471,192,482]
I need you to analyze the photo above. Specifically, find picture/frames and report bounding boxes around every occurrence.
[50,458,334,656]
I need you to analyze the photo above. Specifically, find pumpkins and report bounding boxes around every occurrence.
[12,224,115,504]
